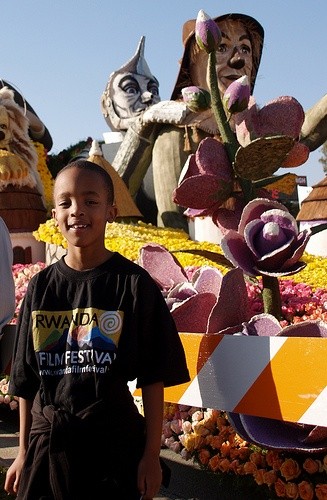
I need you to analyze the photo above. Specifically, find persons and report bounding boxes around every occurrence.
[151,12,327,246]
[99,33,193,234]
[5,160,191,500]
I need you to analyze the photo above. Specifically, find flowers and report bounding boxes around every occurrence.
[138,9,327,452]
